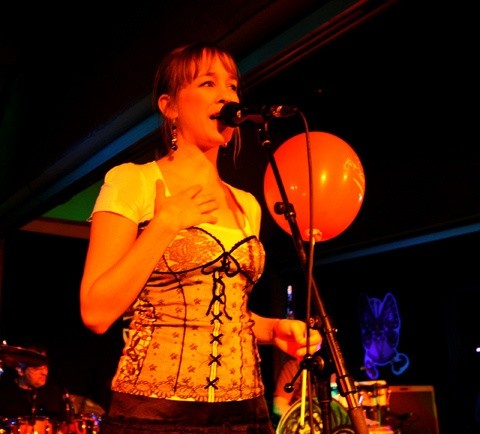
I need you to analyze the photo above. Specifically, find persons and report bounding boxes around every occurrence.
[0,345,70,421]
[273,358,320,416]
[80,41,322,434]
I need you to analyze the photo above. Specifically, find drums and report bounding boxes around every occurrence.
[6,415,54,434]
[67,412,101,434]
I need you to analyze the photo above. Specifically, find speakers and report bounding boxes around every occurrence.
[386,385,439,434]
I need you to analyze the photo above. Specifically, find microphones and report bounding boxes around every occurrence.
[397,412,413,420]
[219,101,299,127]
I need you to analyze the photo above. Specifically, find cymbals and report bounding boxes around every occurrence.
[0,344,48,369]
